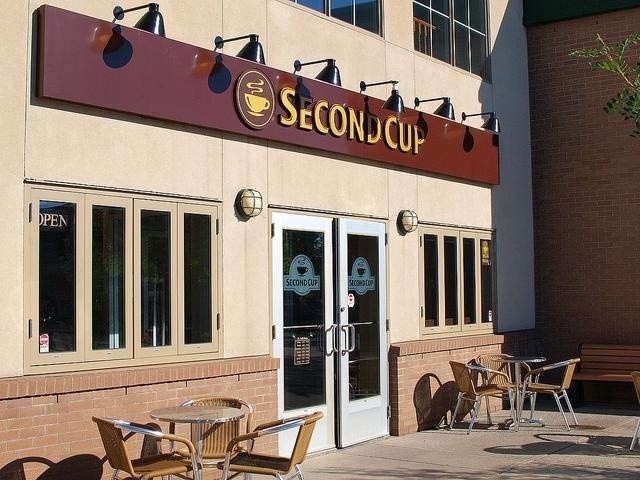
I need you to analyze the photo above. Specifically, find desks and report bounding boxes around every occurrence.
[149,408,245,480]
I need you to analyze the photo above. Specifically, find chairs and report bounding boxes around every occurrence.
[91,415,204,480]
[215,410,324,480]
[411,371,448,431]
[167,396,255,480]
[450,351,583,434]
[628,368,640,452]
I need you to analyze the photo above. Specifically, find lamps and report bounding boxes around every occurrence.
[112,0,167,41]
[291,58,343,90]
[237,188,264,220]
[358,78,405,113]
[413,94,457,121]
[398,209,419,236]
[462,108,500,133]
[211,32,268,65]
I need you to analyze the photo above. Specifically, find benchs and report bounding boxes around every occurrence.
[565,344,640,414]
